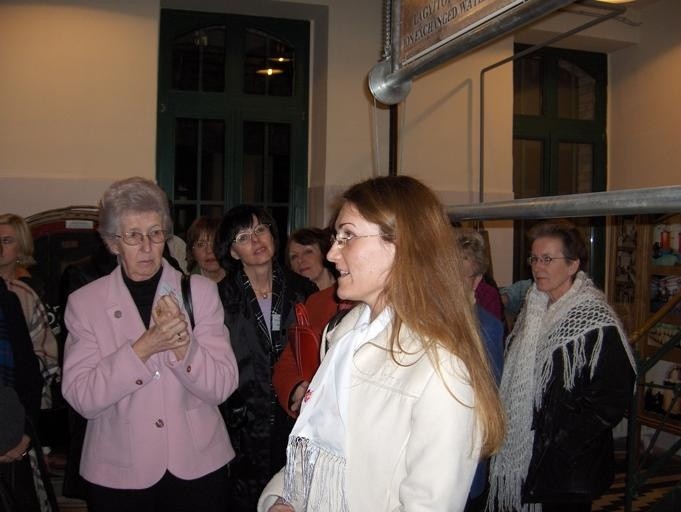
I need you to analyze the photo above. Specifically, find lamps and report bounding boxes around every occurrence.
[255,66,284,76]
[271,54,295,62]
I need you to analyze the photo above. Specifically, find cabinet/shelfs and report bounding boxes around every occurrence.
[608,213,680,436]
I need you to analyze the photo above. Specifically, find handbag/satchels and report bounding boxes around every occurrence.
[221,391,246,464]
[287,303,322,384]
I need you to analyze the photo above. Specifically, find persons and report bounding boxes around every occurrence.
[0,177,636,511]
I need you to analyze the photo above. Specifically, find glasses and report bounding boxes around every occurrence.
[526,255,567,266]
[114,229,170,246]
[329,231,396,248]
[192,240,214,249]
[231,223,273,246]
